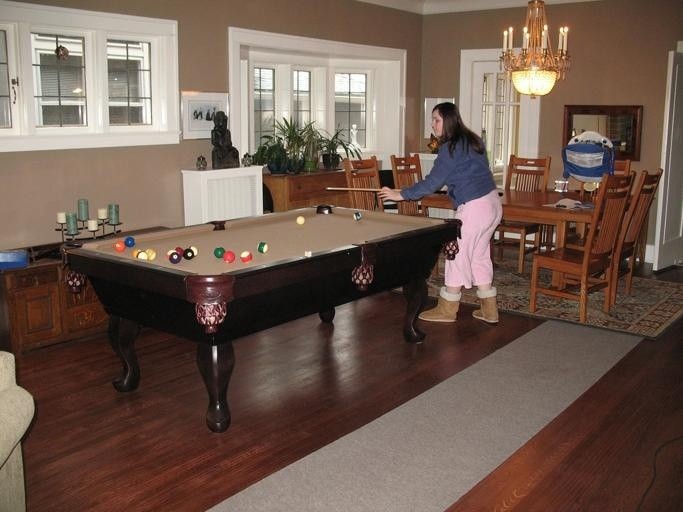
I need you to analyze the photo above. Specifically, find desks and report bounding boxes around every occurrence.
[59,203,463,434]
[262,169,359,212]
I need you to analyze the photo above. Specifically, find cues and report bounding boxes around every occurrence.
[325,186,402,193]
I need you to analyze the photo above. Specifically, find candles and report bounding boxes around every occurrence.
[56,199,120,236]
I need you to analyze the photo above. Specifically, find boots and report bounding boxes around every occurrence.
[418,287,461,323]
[473,288,499,323]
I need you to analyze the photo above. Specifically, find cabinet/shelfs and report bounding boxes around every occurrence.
[2,262,111,360]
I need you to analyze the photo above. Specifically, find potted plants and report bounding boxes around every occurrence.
[259,114,364,174]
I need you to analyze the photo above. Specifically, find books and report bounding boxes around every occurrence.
[544,197,582,209]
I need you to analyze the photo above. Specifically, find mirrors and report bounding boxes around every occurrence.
[563,106,642,161]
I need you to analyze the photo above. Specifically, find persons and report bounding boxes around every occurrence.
[350,123,358,146]
[377,101,506,327]
[210,110,240,169]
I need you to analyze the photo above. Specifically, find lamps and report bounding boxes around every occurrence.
[498,0,573,100]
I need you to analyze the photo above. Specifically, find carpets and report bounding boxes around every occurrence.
[392,254,683,342]
[200,318,646,512]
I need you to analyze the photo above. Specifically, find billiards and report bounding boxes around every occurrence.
[113,235,271,265]
[353,211,361,220]
[296,215,305,225]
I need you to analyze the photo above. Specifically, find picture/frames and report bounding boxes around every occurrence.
[180,90,231,141]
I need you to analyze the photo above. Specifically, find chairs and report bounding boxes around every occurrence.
[0,350,35,512]
